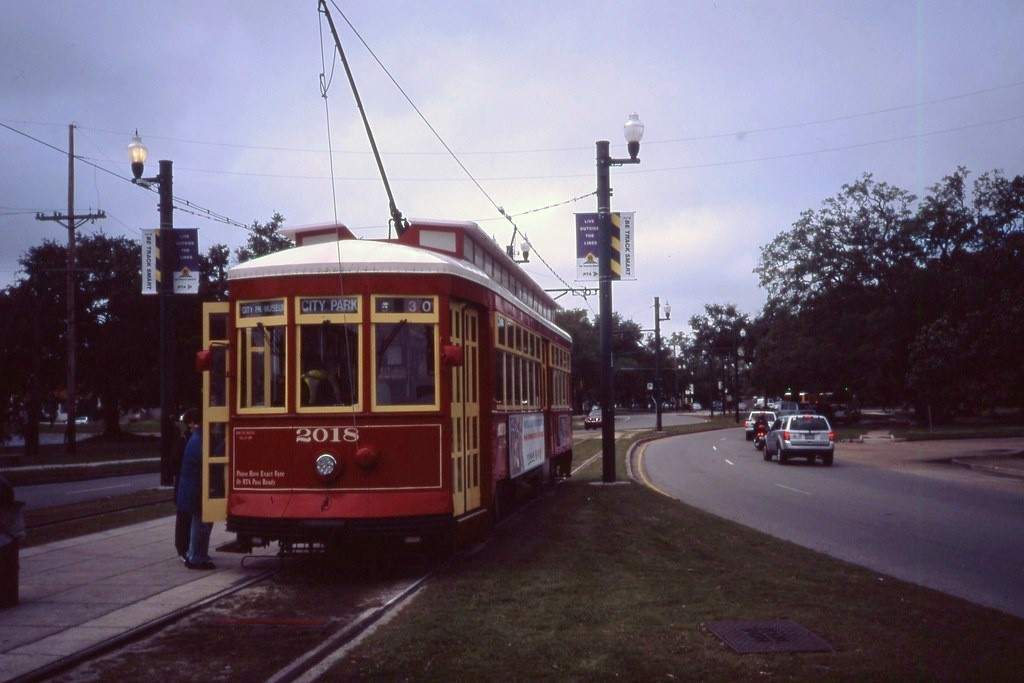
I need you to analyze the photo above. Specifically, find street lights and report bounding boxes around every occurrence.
[733,326,747,424]
[131,128,180,488]
[653,295,671,432]
[596,108,645,485]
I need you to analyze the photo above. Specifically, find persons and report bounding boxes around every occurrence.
[301,359,340,406]
[583,400,592,415]
[175,383,264,569]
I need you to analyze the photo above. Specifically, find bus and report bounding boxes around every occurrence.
[195,215,575,560]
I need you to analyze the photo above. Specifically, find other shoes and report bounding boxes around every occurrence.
[184,559,216,570]
[180,551,188,562]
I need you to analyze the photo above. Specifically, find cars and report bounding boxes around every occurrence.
[688,403,702,410]
[744,398,850,452]
[712,400,724,411]
[584,409,603,429]
[65,416,88,425]
[763,414,835,466]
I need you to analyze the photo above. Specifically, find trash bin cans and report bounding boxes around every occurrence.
[0,476,27,610]
